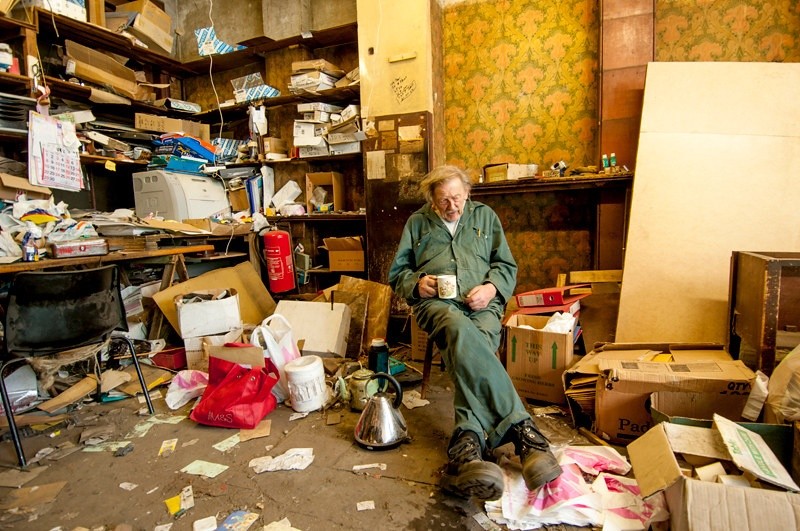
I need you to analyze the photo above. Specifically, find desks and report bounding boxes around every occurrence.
[466,172,634,270]
[0,244,215,341]
[727,251,800,377]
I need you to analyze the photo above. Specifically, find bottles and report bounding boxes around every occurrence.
[601,152,608,167]
[478,175,483,183]
[610,152,616,166]
[368,338,389,392]
[0,43,13,72]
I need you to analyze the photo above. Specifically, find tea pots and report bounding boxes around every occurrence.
[337,368,389,412]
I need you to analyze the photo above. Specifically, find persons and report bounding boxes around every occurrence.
[388,165,564,502]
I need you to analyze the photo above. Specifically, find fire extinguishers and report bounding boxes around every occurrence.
[254,216,295,293]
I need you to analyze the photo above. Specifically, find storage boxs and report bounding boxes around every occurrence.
[61,0,176,101]
[135,112,209,139]
[221,168,303,213]
[271,300,352,357]
[175,288,243,371]
[483,162,538,182]
[290,59,360,91]
[562,340,800,531]
[263,137,285,154]
[323,236,365,271]
[403,313,440,363]
[502,315,574,405]
[0,172,53,203]
[304,172,343,213]
[292,102,366,158]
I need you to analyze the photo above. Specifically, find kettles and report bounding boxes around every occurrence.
[353,372,409,450]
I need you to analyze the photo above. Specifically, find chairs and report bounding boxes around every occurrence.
[0,265,154,467]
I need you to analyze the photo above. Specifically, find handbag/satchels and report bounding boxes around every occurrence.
[249,314,302,403]
[190,342,280,429]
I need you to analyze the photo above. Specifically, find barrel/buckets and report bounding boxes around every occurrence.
[283,354,329,412]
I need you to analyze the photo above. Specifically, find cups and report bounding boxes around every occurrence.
[266,208,276,216]
[434,274,458,299]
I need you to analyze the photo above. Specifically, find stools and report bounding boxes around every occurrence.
[421,334,446,401]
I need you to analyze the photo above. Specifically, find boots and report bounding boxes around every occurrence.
[510,419,563,490]
[443,432,504,502]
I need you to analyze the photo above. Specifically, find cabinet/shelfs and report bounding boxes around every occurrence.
[0,7,367,221]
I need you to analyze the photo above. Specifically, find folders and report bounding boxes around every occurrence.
[516,284,592,308]
